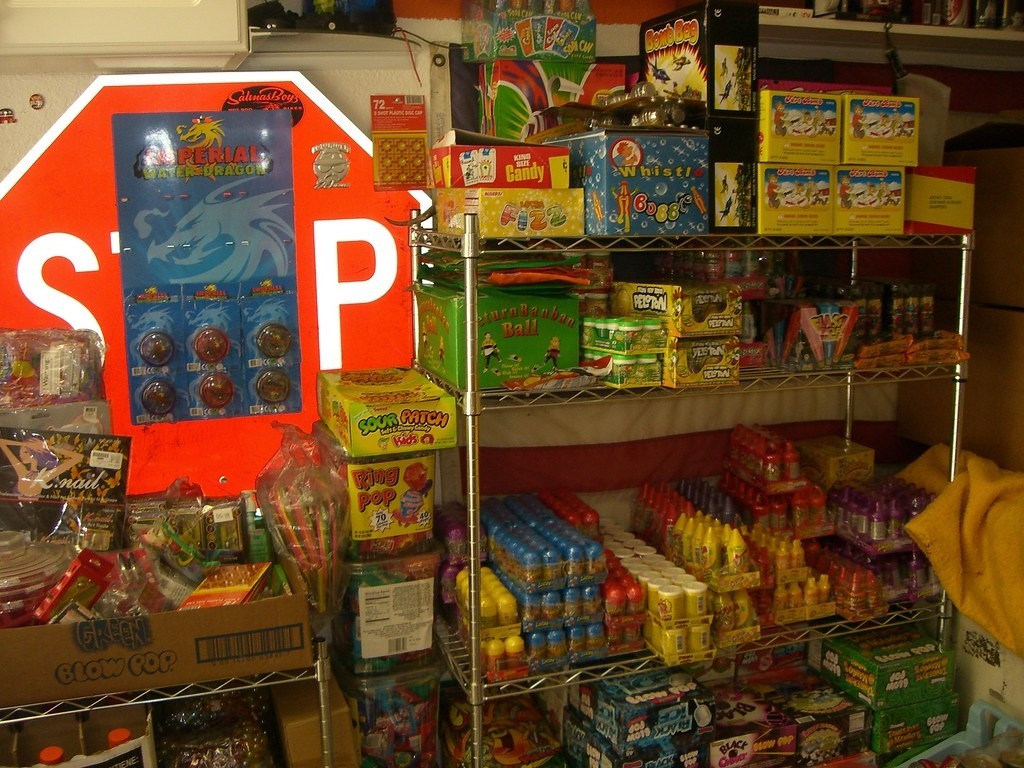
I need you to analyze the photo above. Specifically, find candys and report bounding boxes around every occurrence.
[359,680,438,768]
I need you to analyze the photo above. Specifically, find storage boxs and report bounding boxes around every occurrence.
[0,2,976,768]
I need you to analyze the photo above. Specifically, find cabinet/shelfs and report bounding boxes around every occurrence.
[409,207,974,768]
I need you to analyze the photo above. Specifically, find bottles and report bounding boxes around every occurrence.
[39,747,64,765]
[108,728,131,749]
